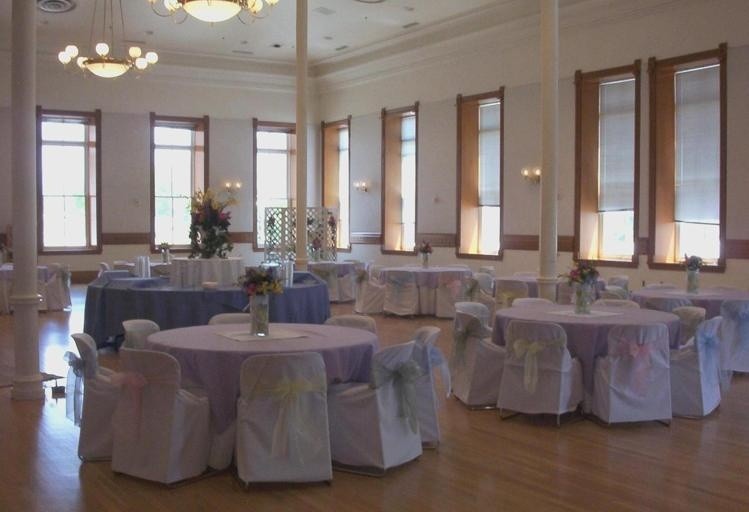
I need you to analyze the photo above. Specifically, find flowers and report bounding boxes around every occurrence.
[186,190,239,260]
[417,239,433,254]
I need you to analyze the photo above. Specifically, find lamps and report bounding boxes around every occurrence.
[355,182,367,193]
[226,182,242,196]
[521,167,540,185]
[58,1,158,78]
[150,0,280,25]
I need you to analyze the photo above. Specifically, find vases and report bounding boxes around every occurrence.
[422,253,429,266]
